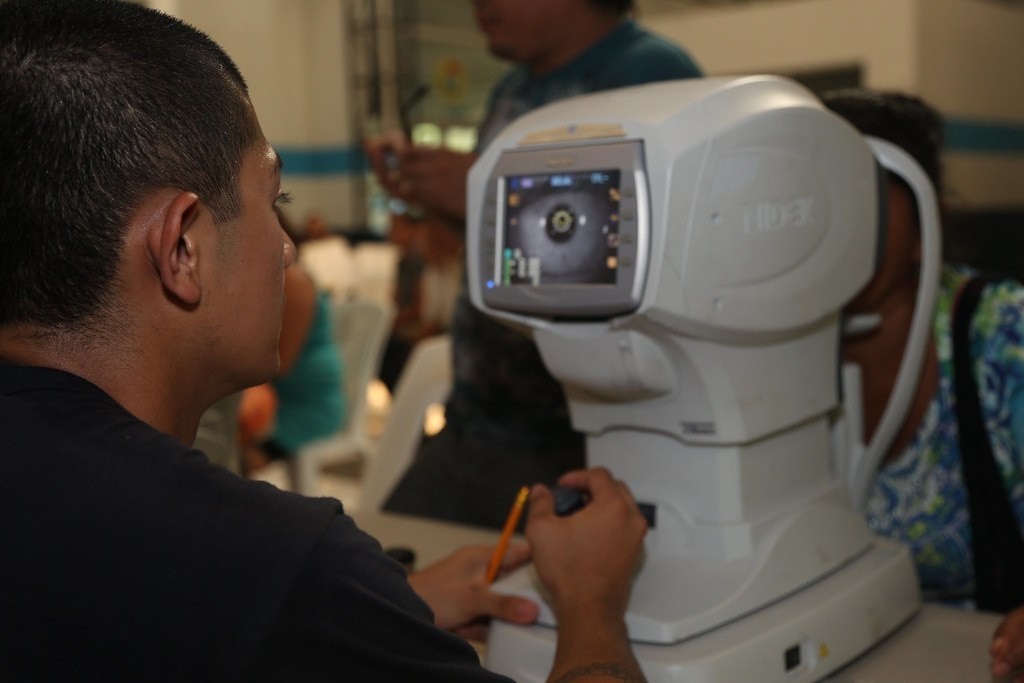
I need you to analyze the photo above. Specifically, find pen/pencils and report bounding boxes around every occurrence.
[487,486,529,585]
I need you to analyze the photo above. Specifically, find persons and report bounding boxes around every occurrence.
[0,0,647,683]
[378,0,708,535]
[815,90,1024,683]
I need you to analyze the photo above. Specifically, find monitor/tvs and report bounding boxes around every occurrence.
[500,168,622,286]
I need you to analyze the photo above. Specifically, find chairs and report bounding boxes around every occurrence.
[261,237,455,512]
